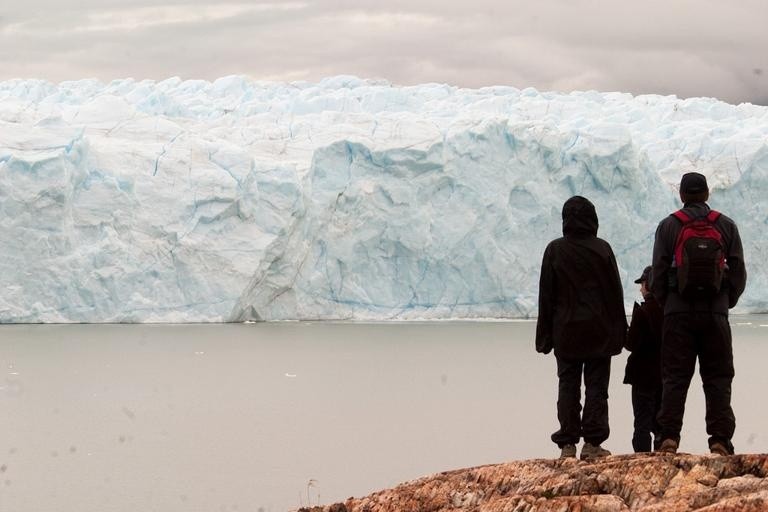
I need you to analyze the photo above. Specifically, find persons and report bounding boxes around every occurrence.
[535,195,629,460]
[623,265,663,452]
[651,172,747,456]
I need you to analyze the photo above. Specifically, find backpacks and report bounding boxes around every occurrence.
[670,211,729,300]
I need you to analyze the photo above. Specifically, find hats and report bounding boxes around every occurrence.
[634,265,652,283]
[680,172,707,195]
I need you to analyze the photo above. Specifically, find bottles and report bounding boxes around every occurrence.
[723,258,729,270]
[672,255,677,267]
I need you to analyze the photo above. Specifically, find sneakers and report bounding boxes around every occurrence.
[659,438,678,452]
[560,444,576,457]
[709,441,731,456]
[580,443,611,459]
